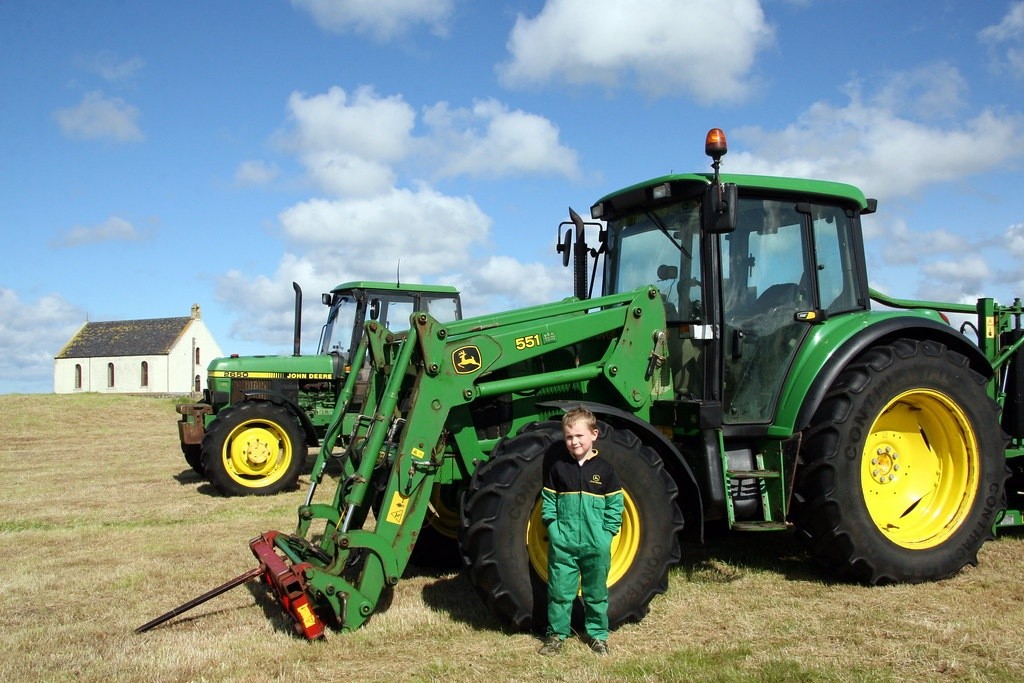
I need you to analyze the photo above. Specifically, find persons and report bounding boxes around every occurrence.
[538,407,624,654]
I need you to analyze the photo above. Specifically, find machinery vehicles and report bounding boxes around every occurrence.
[173,258,464,499]
[130,126,1024,647]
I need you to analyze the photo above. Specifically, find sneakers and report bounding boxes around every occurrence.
[538,634,566,658]
[589,638,611,658]
